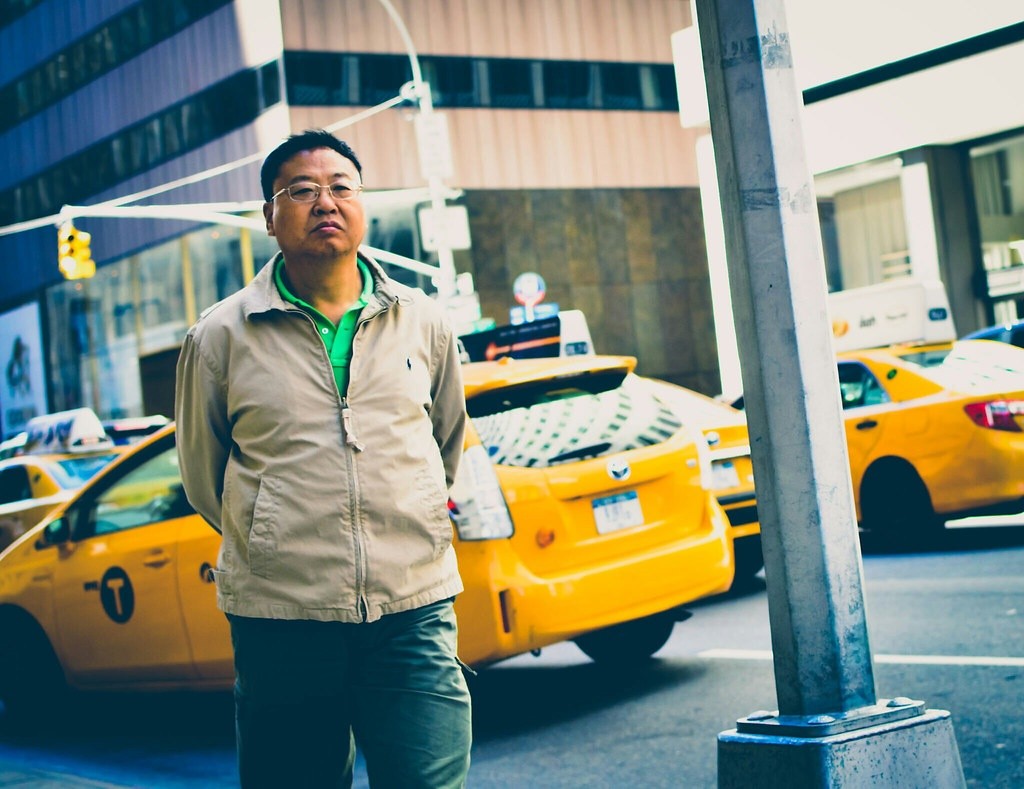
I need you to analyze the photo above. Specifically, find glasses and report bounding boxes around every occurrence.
[269,181,364,203]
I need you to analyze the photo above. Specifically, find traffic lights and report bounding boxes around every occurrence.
[56,223,95,280]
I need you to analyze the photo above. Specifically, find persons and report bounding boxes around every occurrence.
[175,131,471,789]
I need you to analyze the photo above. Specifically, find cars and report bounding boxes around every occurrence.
[0,309,767,580]
[1,354,737,748]
[711,272,1024,550]
